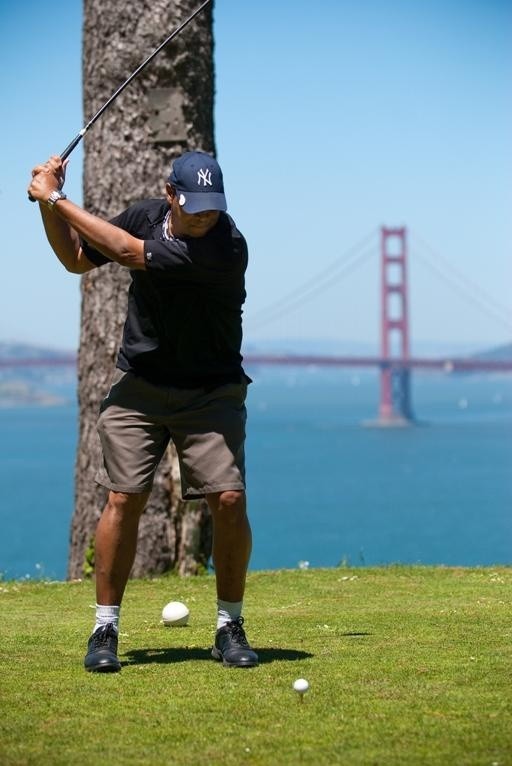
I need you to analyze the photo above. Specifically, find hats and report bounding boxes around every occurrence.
[170,151,227,214]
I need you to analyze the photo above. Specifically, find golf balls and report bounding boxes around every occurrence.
[294,679,309,696]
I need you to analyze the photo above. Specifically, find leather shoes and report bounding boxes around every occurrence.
[212,615,260,667]
[84,622,122,672]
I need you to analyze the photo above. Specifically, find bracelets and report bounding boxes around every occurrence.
[47,191,67,212]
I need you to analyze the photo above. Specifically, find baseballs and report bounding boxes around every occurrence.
[160,601,191,627]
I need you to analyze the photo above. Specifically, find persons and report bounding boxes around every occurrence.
[29,148,260,673]
